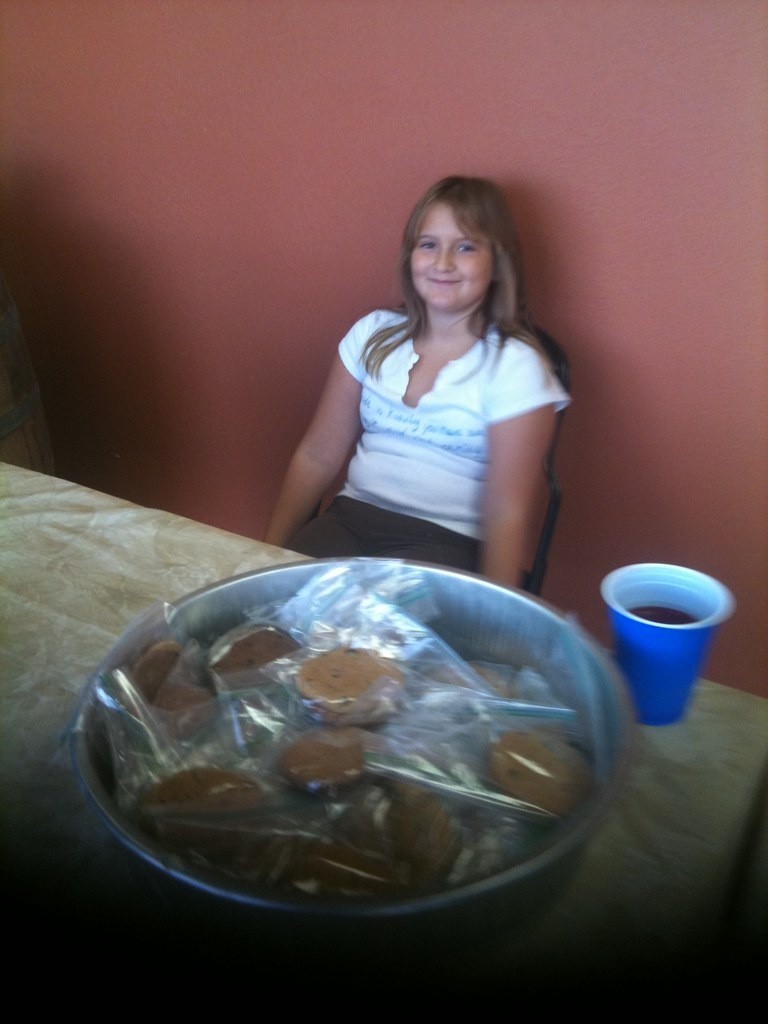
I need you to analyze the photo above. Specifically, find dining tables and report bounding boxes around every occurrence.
[0,460,767,1024]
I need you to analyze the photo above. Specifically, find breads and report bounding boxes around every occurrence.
[129,627,591,892]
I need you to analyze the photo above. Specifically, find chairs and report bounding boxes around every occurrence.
[502,320,570,605]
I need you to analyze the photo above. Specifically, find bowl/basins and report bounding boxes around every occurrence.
[74,549,641,969]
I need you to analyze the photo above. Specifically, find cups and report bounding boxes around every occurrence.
[598,560,730,724]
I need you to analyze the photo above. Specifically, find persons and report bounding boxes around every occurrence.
[265,176,572,595]
